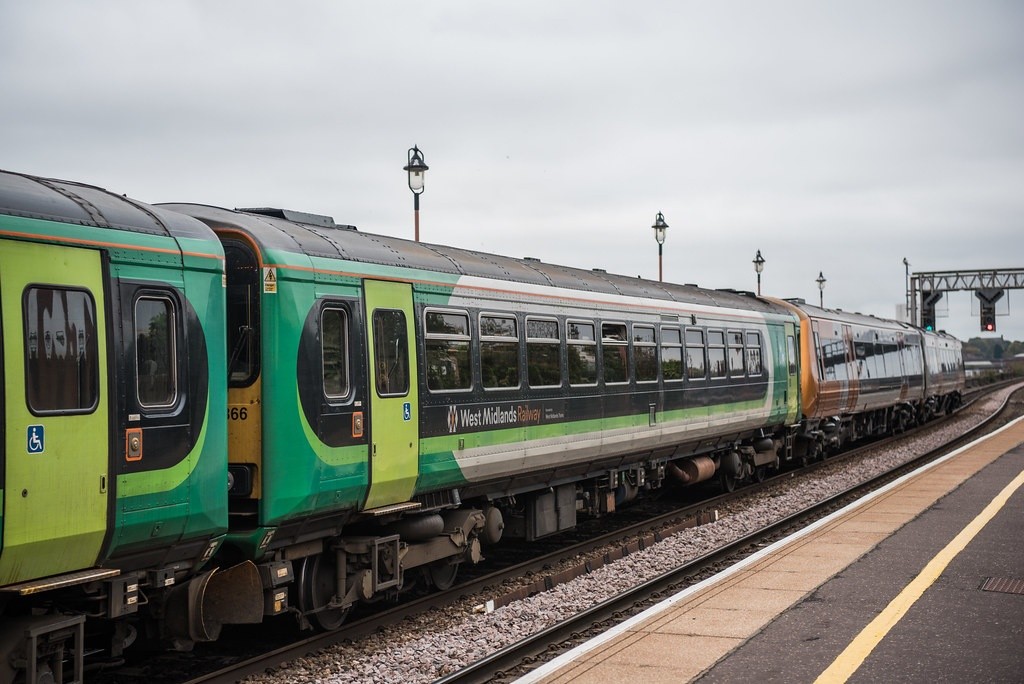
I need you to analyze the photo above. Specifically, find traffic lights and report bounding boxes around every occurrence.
[925,324,933,332]
[986,323,994,331]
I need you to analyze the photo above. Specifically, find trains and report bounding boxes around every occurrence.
[1,167,966,684]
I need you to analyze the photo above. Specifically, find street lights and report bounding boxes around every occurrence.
[651,210,670,281]
[814,270,827,309]
[402,143,430,243]
[751,248,768,297]
